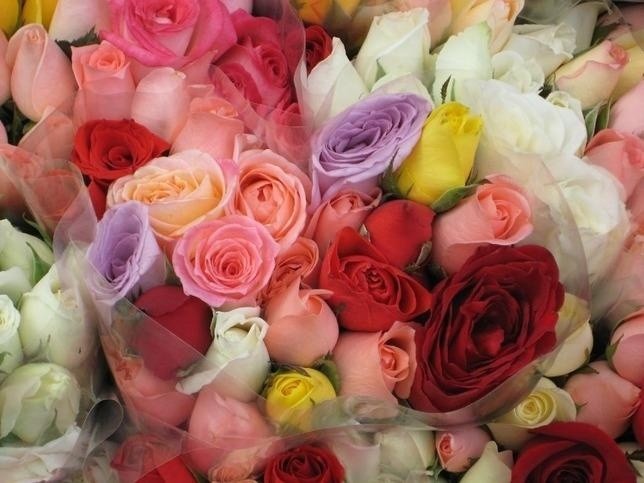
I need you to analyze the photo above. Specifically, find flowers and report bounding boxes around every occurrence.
[0,1,644,483]
